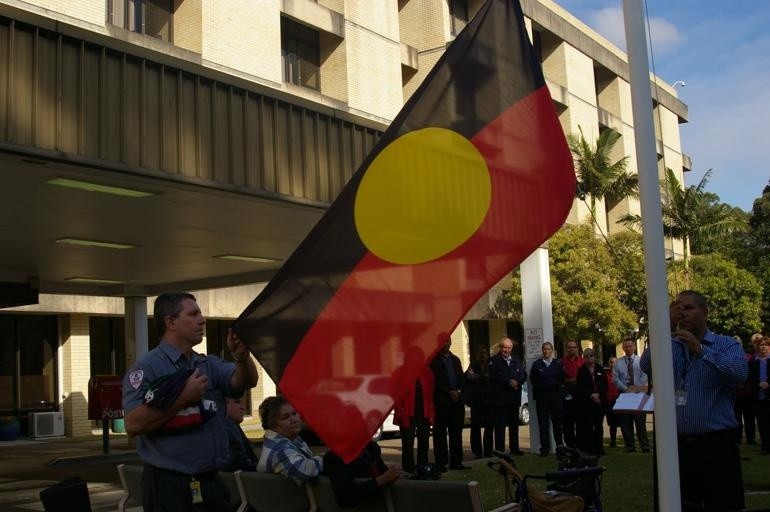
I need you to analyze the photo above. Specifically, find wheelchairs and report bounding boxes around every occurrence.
[484,444,608,512]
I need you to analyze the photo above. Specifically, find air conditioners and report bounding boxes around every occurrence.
[28,411,64,437]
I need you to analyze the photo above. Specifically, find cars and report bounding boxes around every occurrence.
[452,366,530,432]
[302,370,445,448]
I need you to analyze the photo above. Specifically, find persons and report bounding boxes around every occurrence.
[323,441,401,512]
[122,292,258,512]
[639,290,750,512]
[389,332,650,474]
[732,334,770,455]
[224,390,259,472]
[256,396,327,487]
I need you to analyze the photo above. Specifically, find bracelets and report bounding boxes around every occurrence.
[700,344,703,350]
[232,351,250,363]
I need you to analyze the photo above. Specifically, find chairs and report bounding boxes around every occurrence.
[40,477,92,512]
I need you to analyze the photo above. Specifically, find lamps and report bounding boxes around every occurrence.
[672,80,686,88]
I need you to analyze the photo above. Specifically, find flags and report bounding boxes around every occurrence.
[231,0,576,464]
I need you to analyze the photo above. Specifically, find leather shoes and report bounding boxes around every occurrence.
[450,463,472,470]
[511,450,524,455]
[642,446,650,452]
[627,446,635,451]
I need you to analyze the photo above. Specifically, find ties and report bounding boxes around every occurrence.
[628,358,634,386]
